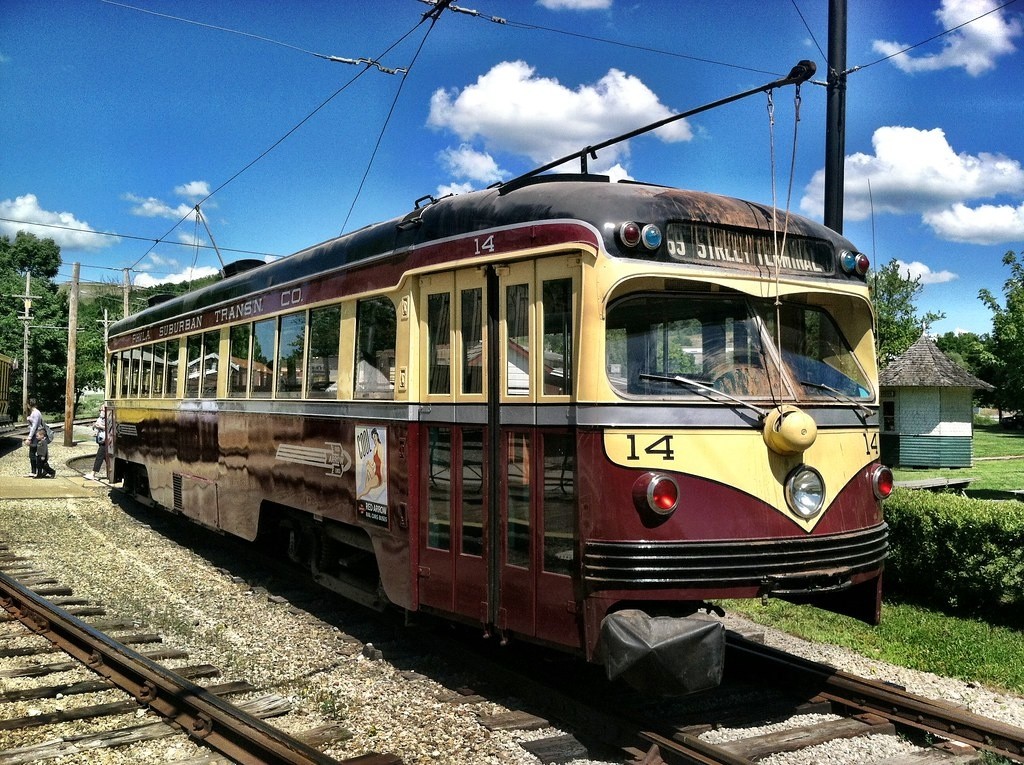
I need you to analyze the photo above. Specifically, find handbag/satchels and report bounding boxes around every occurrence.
[95,429,106,444]
[41,419,54,444]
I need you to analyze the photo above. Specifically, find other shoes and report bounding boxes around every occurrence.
[84,474,95,479]
[51,470,56,478]
[42,471,48,476]
[24,473,38,477]
[34,476,41,479]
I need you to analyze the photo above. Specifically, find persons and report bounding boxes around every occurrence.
[23,397,51,477]
[83,403,107,480]
[27,429,57,479]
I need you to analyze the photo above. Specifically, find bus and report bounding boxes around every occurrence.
[104,59,894,700]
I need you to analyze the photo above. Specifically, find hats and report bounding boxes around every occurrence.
[99,404,106,410]
